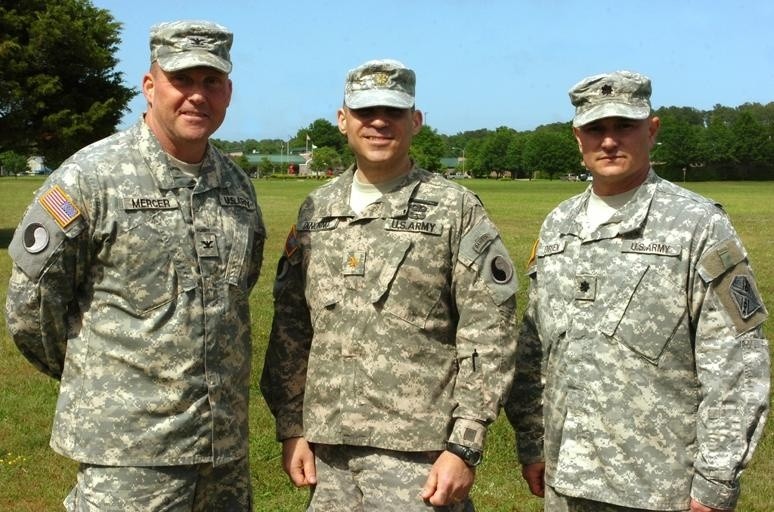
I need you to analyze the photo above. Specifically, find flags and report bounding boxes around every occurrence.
[306,134,310,141]
[311,143,317,150]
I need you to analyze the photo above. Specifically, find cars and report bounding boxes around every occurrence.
[566,173,595,182]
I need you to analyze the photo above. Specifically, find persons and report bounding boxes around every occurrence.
[500,69,772,511]
[258,59,519,510]
[4,19,269,511]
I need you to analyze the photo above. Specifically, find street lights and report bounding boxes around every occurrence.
[450,146,465,175]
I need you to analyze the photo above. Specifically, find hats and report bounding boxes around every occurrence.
[344,60,414,110]
[151,21,233,73]
[569,71,651,128]
